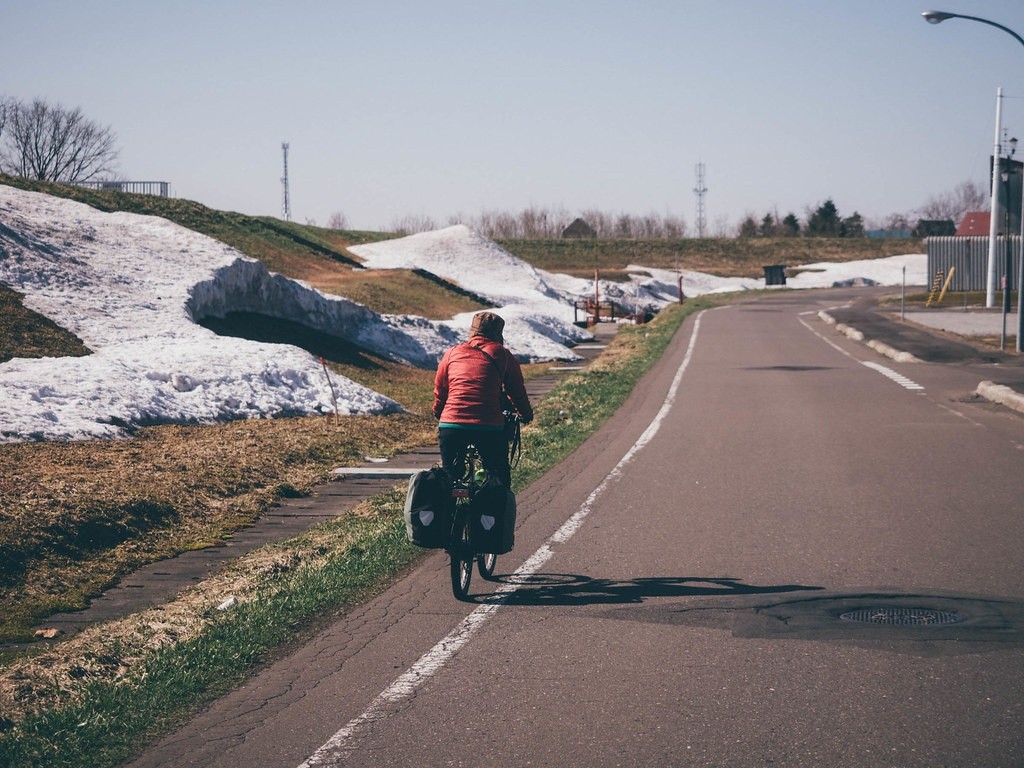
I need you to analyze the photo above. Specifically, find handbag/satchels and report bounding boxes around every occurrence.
[473,473,516,553]
[500,390,518,440]
[404,466,453,548]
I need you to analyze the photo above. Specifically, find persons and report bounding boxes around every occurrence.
[432,312,533,490]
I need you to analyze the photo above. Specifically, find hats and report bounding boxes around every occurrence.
[469,311,505,345]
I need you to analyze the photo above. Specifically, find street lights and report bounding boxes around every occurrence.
[996,136,1019,315]
[921,10,1023,353]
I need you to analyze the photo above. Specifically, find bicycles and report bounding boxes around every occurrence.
[447,409,525,599]
